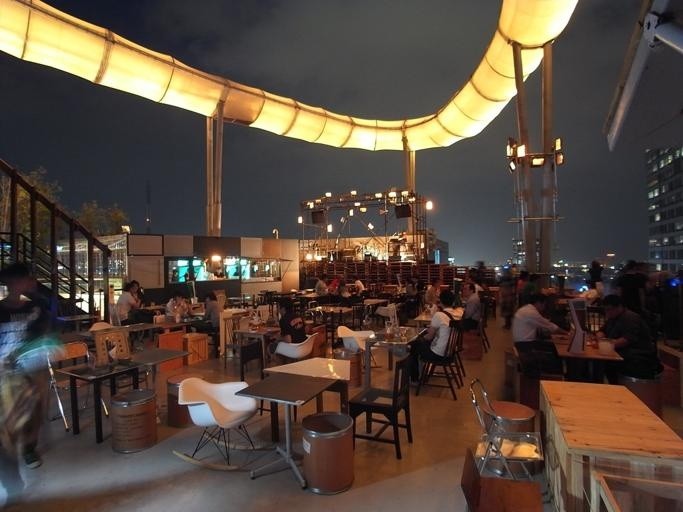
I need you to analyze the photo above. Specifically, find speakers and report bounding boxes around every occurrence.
[394,204,411,219]
[311,210,326,224]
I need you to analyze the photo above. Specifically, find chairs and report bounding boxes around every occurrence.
[470,379,543,481]
[585,306,605,331]
[46,343,110,432]
[84,281,492,402]
[350,353,413,462]
[172,374,267,473]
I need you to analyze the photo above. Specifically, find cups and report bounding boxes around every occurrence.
[596,337,616,354]
[84,346,116,368]
[384,320,407,337]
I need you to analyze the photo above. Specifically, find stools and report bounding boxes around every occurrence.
[489,401,533,474]
[112,387,158,453]
[618,372,662,418]
[299,410,357,495]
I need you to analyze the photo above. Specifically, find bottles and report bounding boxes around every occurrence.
[175,308,180,323]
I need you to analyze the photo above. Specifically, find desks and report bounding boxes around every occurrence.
[591,473,683,512]
[262,355,349,446]
[233,372,340,490]
[539,380,683,512]
[551,335,623,380]
[57,362,147,443]
[114,348,192,427]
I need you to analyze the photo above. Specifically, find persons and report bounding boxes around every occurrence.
[425,260,496,330]
[1,259,73,469]
[614,260,683,338]
[499,264,565,330]
[166,292,219,333]
[587,260,601,289]
[233,264,270,277]
[267,297,306,362]
[408,290,464,385]
[172,267,224,281]
[511,294,574,378]
[596,295,664,384]
[300,267,424,326]
[117,280,150,343]
